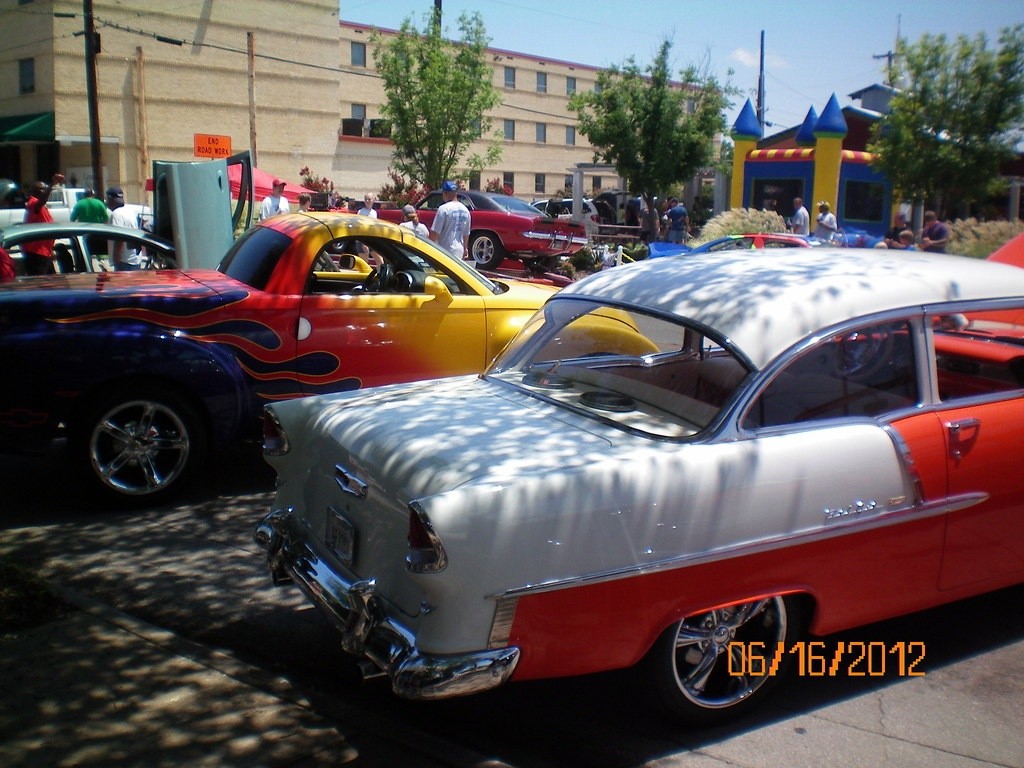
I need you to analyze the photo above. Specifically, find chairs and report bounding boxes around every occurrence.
[776,374,905,419]
[687,355,762,414]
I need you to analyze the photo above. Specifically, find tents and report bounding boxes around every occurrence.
[140,165,314,229]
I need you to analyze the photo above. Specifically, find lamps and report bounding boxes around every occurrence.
[493,52,504,63]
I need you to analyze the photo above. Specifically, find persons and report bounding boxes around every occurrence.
[623,195,691,251]
[259,180,289,220]
[70,186,150,270]
[791,198,809,236]
[814,203,837,240]
[921,211,948,253]
[20,175,64,275]
[329,192,382,271]
[400,206,429,265]
[298,195,310,213]
[875,212,916,251]
[430,181,471,260]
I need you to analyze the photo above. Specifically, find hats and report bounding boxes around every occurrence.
[272,179,286,187]
[817,201,830,210]
[403,205,416,215]
[106,187,124,199]
[443,180,457,191]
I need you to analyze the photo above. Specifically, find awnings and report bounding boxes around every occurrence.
[0,112,55,141]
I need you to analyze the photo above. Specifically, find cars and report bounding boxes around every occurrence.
[0,222,177,277]
[646,233,845,259]
[528,189,633,235]
[0,212,661,504]
[375,190,589,270]
[257,233,1024,727]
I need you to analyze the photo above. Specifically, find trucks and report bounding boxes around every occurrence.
[0,184,113,231]
